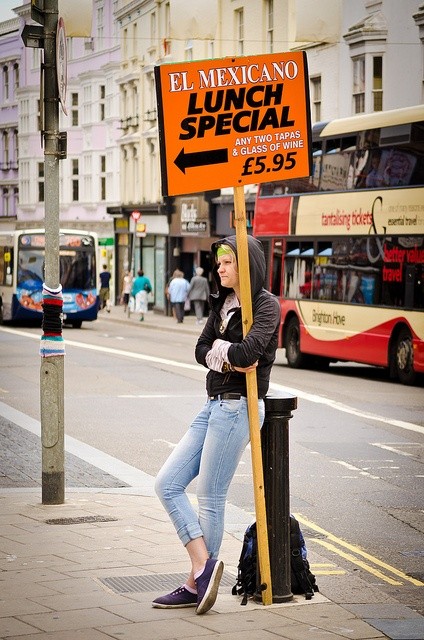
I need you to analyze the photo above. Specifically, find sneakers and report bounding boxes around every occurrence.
[151,586,200,610]
[192,559,223,614]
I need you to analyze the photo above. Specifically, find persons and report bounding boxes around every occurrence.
[122,271,133,319]
[168,271,190,323]
[166,268,179,321]
[151,233,281,614]
[99,265,112,312]
[131,270,153,322]
[189,267,210,325]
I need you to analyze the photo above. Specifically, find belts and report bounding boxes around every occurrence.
[208,392,240,400]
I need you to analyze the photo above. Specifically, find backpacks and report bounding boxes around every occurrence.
[231,514,319,606]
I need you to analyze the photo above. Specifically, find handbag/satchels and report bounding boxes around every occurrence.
[144,283,152,294]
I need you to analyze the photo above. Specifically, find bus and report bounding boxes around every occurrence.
[0,227,99,328]
[251,105,424,383]
